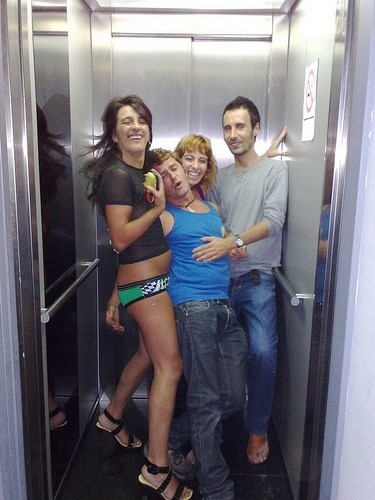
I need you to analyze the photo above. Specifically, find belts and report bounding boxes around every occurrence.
[214,299,229,308]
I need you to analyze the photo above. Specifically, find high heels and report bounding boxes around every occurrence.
[137,457,193,500]
[95,407,143,449]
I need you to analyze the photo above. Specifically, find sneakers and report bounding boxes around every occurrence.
[143,439,196,481]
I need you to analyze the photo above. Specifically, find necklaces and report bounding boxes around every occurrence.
[177,197,196,213]
[191,185,194,190]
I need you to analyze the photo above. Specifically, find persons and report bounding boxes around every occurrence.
[187,95,288,465]
[34,99,72,431]
[75,95,193,500]
[175,126,288,200]
[106,148,249,500]
[303,151,335,468]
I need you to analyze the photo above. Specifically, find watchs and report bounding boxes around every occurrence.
[234,233,243,248]
